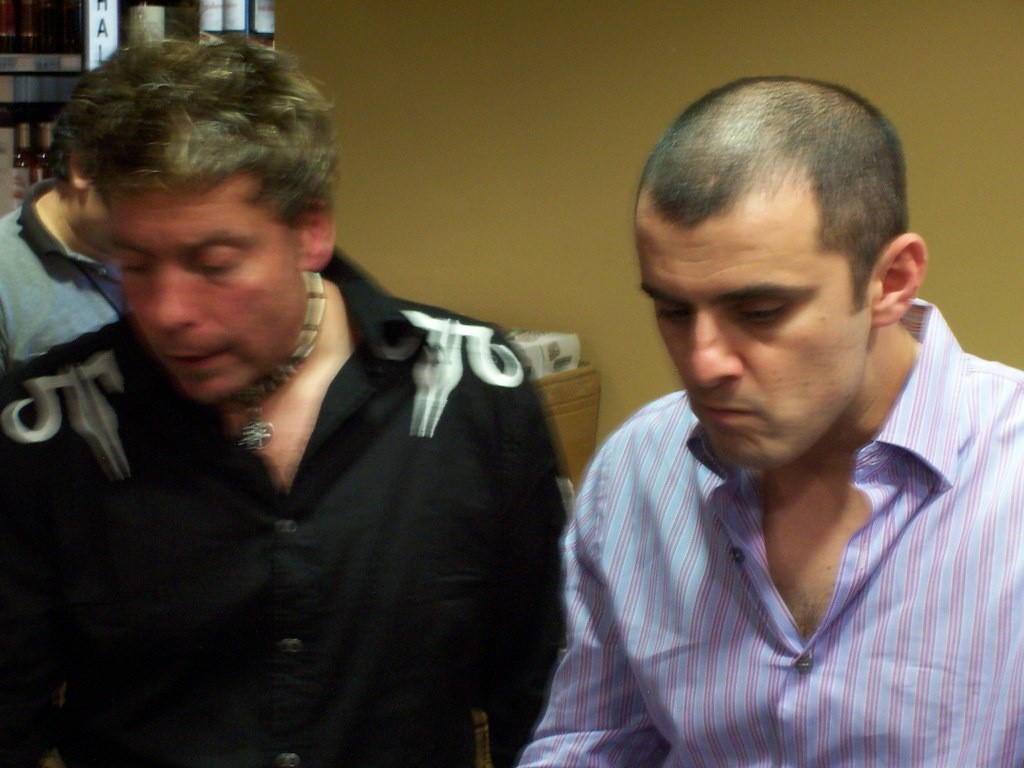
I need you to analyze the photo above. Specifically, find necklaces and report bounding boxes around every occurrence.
[218,270,326,452]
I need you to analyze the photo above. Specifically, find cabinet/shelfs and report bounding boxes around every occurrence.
[527,359,602,522]
[0,0,118,219]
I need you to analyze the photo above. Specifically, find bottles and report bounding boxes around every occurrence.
[35,123,55,182]
[14,124,33,197]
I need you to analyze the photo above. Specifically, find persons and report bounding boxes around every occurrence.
[1,61,124,367]
[518,78,1024,767]
[0,33,567,767]
